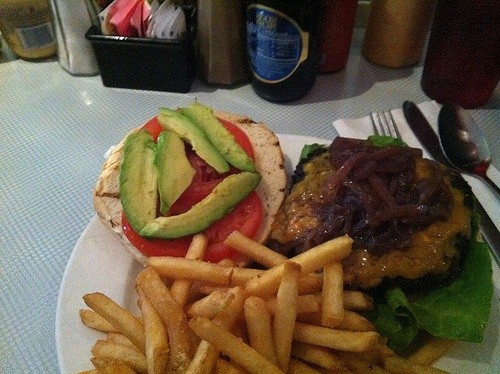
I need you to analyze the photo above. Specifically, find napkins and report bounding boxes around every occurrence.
[332,99,500,275]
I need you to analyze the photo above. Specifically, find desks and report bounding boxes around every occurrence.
[1,30,500,373]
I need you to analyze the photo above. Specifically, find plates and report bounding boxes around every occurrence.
[54,134,500,374]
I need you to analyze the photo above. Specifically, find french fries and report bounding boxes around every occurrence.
[78,229,456,374]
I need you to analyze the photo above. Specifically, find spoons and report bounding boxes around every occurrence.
[436,101,500,202]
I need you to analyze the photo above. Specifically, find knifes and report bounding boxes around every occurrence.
[402,97,500,264]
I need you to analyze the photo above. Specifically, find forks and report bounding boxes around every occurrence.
[369,108,402,142]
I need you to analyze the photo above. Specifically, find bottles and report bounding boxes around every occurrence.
[193,0,250,90]
[0,0,56,61]
[244,0,328,106]
[48,0,103,77]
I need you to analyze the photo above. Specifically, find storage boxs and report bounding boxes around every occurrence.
[84,5,197,93]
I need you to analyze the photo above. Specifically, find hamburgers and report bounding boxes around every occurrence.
[90,102,493,350]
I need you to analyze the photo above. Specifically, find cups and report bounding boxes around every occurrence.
[363,0,434,70]
[423,0,500,111]
[318,0,359,74]
